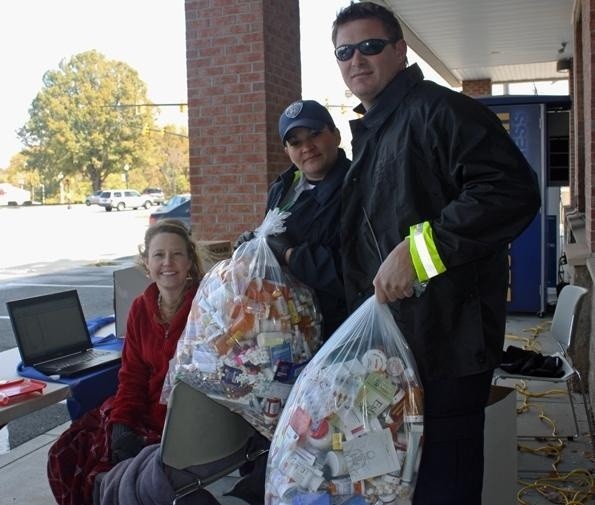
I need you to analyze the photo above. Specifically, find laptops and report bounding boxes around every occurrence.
[6,287,122,380]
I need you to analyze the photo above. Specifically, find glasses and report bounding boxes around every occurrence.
[334,38,391,62]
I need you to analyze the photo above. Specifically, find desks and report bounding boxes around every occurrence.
[0,320,152,436]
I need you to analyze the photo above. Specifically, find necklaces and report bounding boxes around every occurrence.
[157,293,183,325]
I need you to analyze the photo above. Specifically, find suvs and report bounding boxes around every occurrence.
[96,189,154,211]
[142,185,165,205]
[85,190,103,206]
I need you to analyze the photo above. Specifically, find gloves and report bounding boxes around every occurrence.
[267,237,292,262]
[232,230,254,250]
[109,424,138,462]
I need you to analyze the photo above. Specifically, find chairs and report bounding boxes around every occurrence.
[92,379,271,504]
[490,284,595,442]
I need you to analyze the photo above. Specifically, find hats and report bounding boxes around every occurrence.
[277,100,329,146]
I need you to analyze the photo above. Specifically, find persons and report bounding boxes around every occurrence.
[232,99,353,349]
[330,2,543,505]
[45,218,204,505]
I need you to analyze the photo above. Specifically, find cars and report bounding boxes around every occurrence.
[149,198,191,234]
